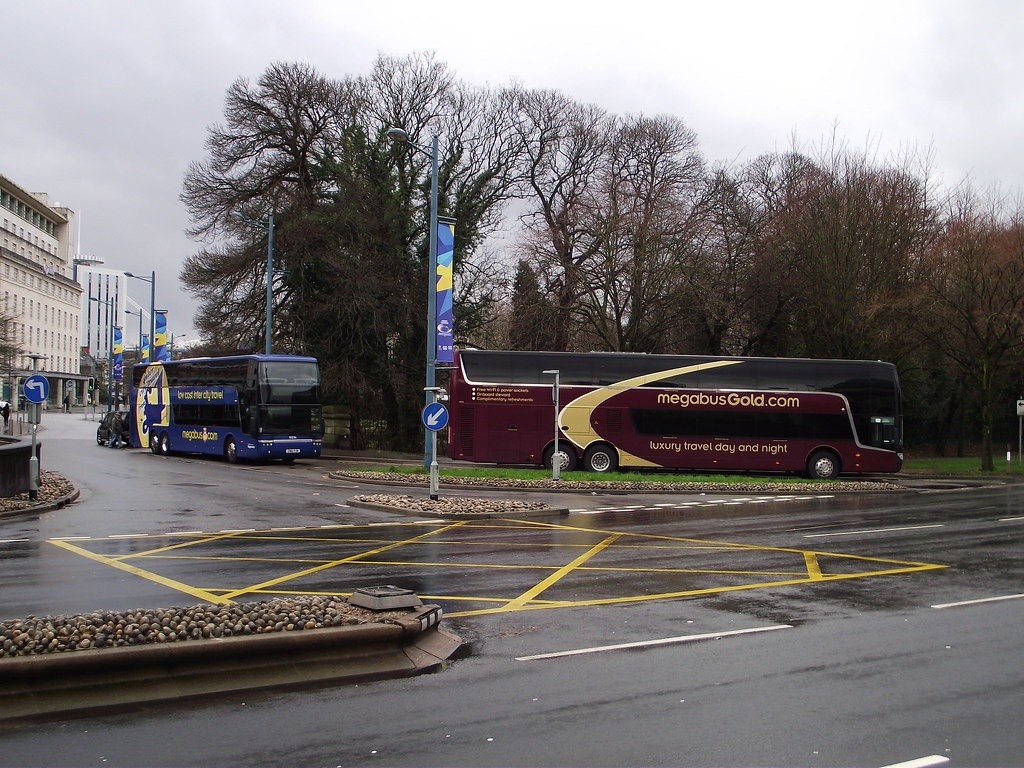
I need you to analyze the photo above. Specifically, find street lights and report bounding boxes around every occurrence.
[89,297,114,412]
[125,309,142,363]
[542,369,561,481]
[232,210,274,356]
[124,270,155,363]
[385,127,440,475]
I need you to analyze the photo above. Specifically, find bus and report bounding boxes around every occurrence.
[445,344,906,480]
[129,354,326,465]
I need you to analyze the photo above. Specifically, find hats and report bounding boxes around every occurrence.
[116,413,121,415]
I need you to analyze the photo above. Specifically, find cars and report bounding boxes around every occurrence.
[96,410,131,445]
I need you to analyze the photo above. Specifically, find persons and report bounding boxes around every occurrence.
[64,396,70,410]
[0,403,9,425]
[107,413,124,449]
